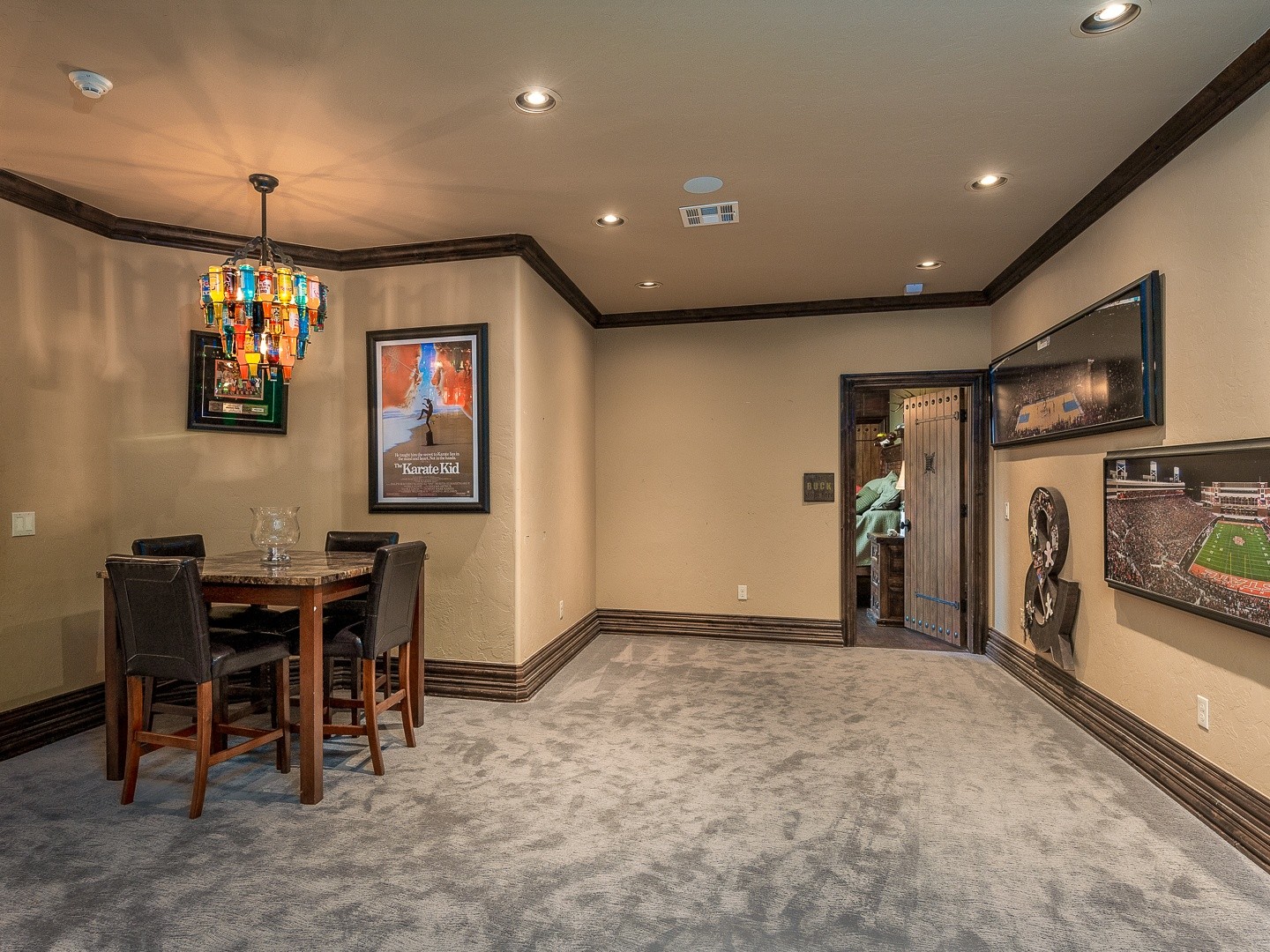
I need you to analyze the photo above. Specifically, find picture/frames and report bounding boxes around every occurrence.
[1104,437,1270,636]
[989,270,1164,449]
[366,322,491,514]
[187,329,288,435]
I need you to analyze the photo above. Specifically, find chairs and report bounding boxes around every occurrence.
[105,553,290,819]
[275,541,426,775]
[272,531,399,738]
[132,534,280,759]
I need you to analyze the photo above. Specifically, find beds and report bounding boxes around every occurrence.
[856,443,901,576]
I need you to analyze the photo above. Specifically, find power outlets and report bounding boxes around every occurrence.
[1197,695,1209,730]
[559,600,564,619]
[738,585,747,600]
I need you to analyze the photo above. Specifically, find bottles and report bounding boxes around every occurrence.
[195,263,328,383]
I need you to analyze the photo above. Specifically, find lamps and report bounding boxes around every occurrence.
[199,173,327,384]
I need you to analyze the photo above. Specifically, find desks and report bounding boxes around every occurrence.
[95,550,429,805]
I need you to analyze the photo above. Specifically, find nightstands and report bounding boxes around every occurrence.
[867,532,904,628]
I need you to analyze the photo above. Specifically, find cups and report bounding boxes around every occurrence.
[247,507,302,564]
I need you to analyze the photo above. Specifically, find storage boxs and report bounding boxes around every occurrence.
[250,507,301,565]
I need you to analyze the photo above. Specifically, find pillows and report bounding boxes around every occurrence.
[870,470,900,511]
[857,486,879,514]
